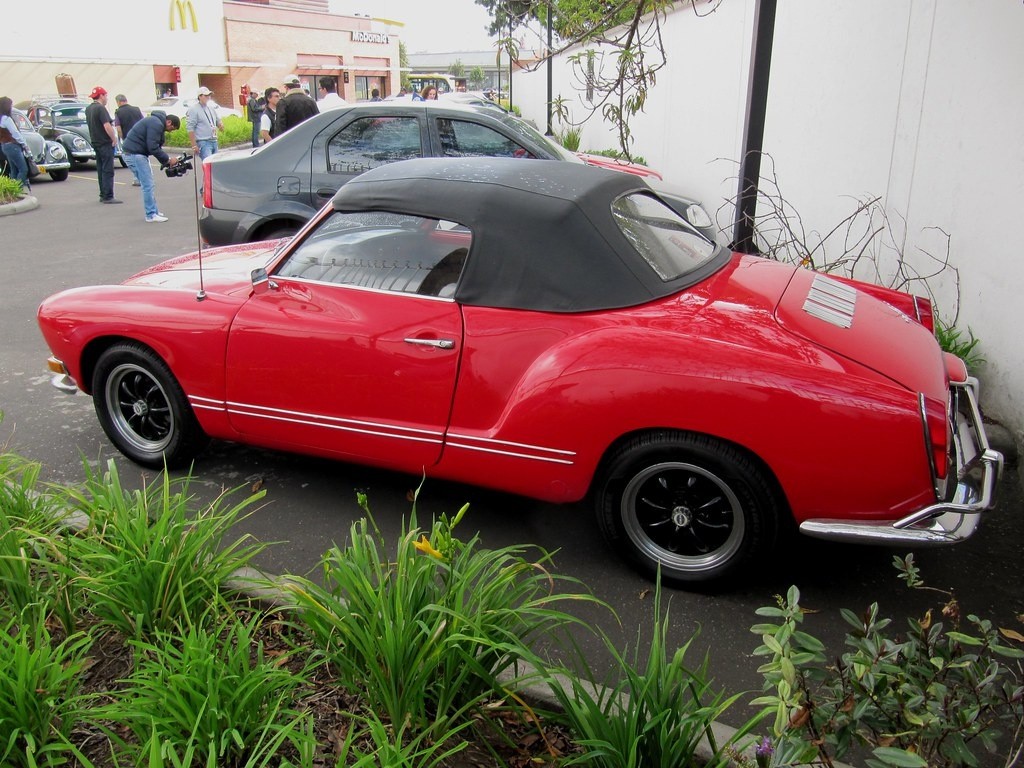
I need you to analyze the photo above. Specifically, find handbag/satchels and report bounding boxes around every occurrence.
[22,153,40,177]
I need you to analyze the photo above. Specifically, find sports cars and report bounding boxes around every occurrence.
[36,152,1004,598]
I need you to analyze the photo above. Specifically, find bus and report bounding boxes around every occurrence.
[407,73,456,94]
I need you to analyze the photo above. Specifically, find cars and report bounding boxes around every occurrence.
[10,108,71,182]
[437,91,540,131]
[138,96,243,124]
[199,101,717,246]
[13,96,128,173]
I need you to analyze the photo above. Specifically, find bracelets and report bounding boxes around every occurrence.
[191,146,197,149]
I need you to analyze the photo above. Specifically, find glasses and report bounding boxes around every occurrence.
[317,87,323,90]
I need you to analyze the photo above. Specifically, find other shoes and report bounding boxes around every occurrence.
[146,213,168,223]
[157,212,164,216]
[99,197,123,204]
[132,180,141,185]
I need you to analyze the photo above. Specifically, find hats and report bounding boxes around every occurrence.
[283,74,301,85]
[250,88,261,96]
[167,88,172,92]
[89,87,107,98]
[197,87,212,95]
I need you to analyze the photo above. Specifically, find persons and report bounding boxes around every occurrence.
[162,87,175,98]
[0,96,31,189]
[412,85,439,102]
[84,86,117,205]
[115,94,144,187]
[246,75,353,148]
[185,86,225,197]
[120,109,181,224]
[369,88,384,102]
[397,86,408,98]
